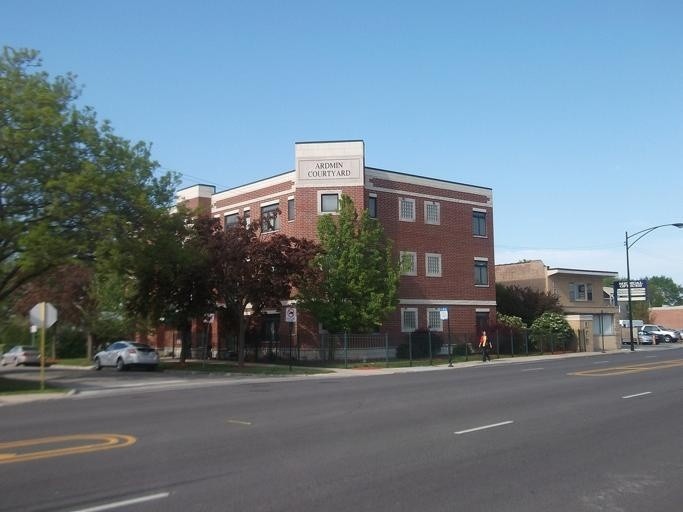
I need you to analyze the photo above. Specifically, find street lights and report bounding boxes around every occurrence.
[625,223,683,351]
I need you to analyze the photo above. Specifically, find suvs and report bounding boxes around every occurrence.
[640,323,679,343]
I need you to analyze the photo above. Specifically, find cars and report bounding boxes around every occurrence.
[677,329,683,340]
[638,329,659,344]
[0,345,47,366]
[92,340,158,371]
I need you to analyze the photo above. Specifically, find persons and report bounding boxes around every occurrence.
[478,328,493,363]
